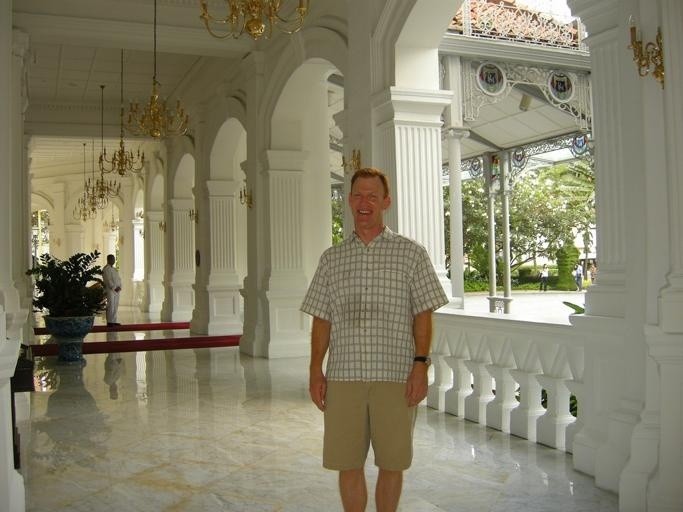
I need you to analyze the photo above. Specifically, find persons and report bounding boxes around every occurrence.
[300,167,448,512]
[590,268,593,282]
[538,264,549,294]
[576,263,582,292]
[104,327,127,401]
[574,265,581,291]
[102,255,123,328]
[591,265,596,272]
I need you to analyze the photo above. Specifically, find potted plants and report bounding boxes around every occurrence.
[25,250,110,366]
[29,363,113,474]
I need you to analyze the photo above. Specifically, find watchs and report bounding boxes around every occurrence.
[414,357,432,367]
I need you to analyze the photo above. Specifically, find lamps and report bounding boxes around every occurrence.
[240,186,253,209]
[140,229,146,239]
[72,0,190,222]
[159,221,166,232]
[520,94,533,112]
[188,209,198,223]
[200,0,310,41]
[340,149,361,174]
[628,15,664,90]
